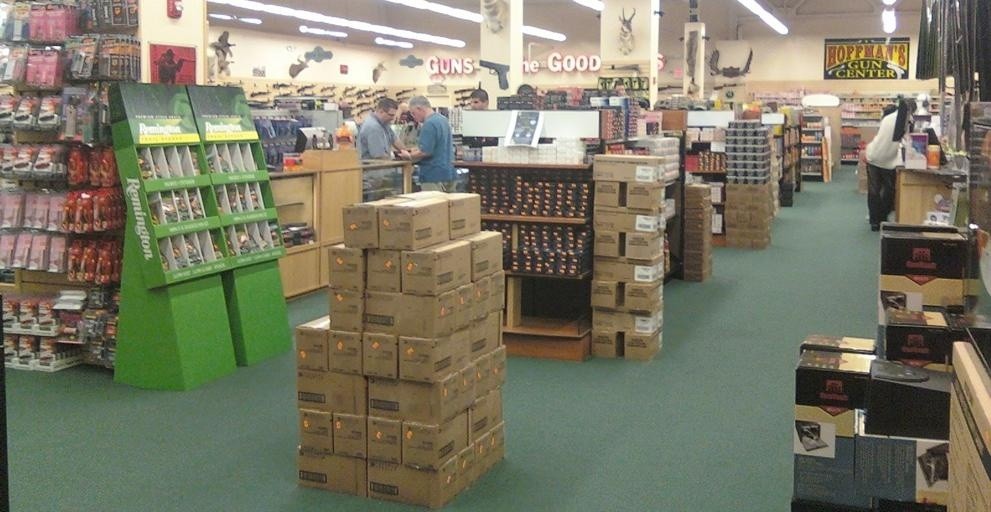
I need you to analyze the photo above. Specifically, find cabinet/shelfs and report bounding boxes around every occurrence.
[452,161,593,362]
[109,81,293,390]
[276,148,412,301]
[774,96,953,183]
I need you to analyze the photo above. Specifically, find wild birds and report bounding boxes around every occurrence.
[210,31,236,57]
[217,50,235,77]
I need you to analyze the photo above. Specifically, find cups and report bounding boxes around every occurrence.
[926,144,941,170]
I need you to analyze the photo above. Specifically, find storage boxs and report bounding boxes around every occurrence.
[790,222,967,512]
[591,156,773,360]
[328,191,506,339]
[295,309,506,511]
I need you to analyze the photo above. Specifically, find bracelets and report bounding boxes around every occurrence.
[407,153,411,162]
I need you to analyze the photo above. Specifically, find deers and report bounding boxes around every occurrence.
[289,55,310,79]
[616,7,637,56]
[373,59,387,83]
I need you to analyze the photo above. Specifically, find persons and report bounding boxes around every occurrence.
[615,80,626,97]
[469,89,489,110]
[397,94,454,192]
[865,97,917,229]
[356,97,415,159]
[517,84,534,95]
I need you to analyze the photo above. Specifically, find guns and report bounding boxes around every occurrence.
[479,60,510,90]
